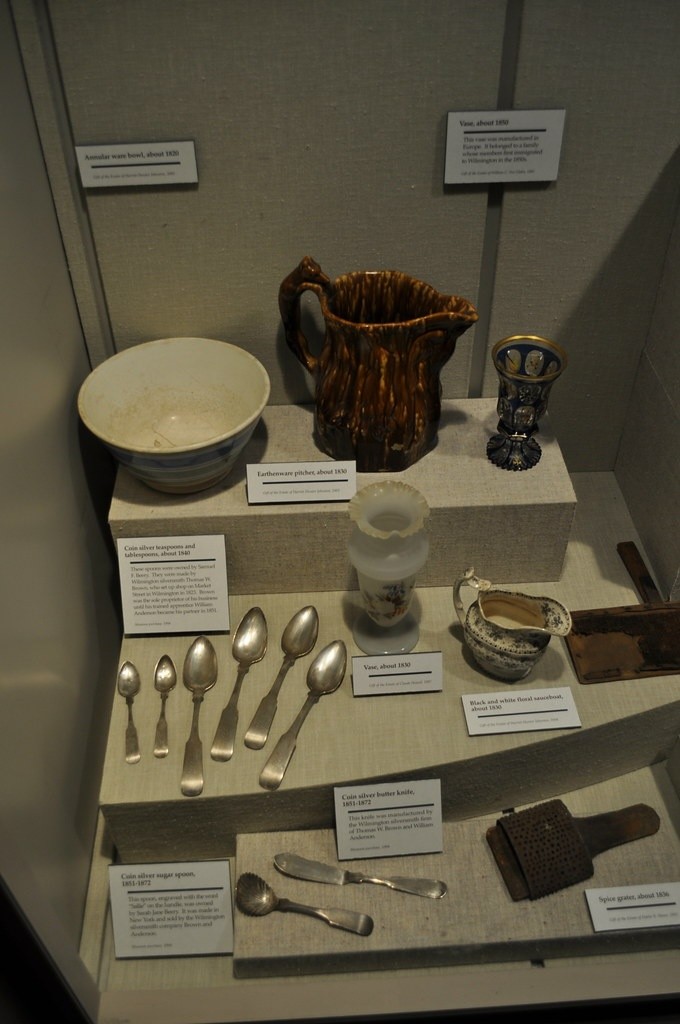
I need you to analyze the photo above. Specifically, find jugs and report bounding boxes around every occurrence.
[277,255,479,475]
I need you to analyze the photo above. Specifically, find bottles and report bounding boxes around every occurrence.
[350,480,431,657]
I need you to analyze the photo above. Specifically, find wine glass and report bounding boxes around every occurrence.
[486,335,567,472]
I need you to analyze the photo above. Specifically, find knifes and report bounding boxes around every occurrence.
[274,852,448,899]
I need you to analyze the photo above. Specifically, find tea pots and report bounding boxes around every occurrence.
[453,565,570,682]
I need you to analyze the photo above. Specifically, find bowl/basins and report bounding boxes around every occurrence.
[76,337,270,495]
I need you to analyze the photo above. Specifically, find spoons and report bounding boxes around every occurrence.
[210,606,269,762]
[180,636,219,797]
[151,654,176,758]
[259,639,347,790]
[117,660,143,765]
[235,872,374,936]
[244,604,321,750]
